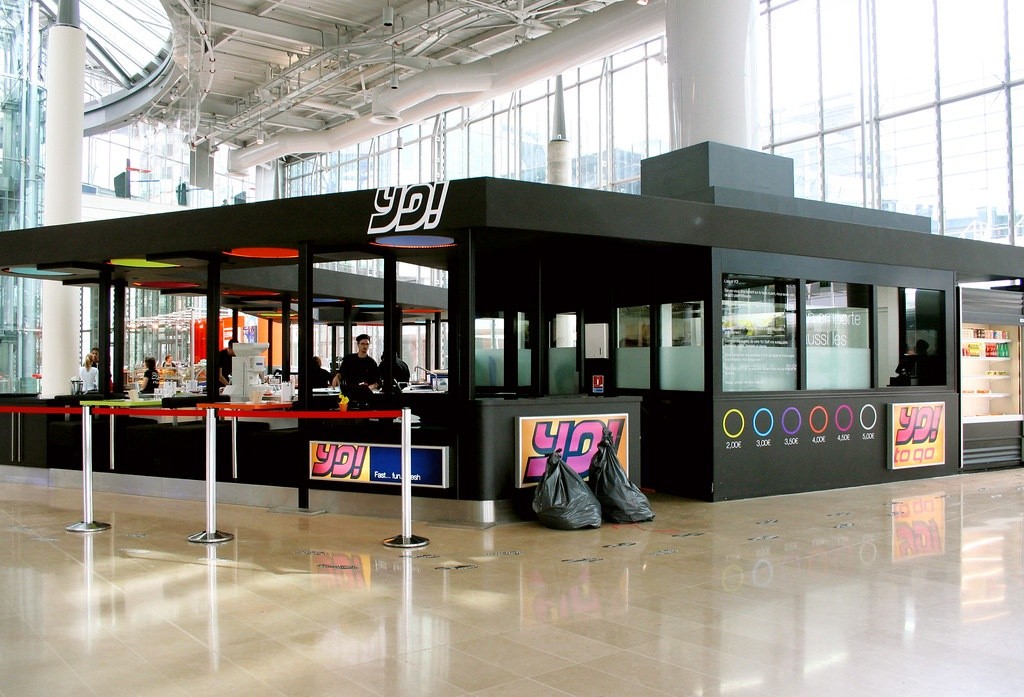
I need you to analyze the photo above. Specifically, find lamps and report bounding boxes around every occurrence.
[391,50,398,90]
[383,0,393,26]
[257,109,264,145]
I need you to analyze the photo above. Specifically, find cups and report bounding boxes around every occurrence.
[339,404,348,411]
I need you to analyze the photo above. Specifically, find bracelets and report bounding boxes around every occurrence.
[337,374,341,379]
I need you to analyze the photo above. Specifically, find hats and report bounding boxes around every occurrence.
[356,333,370,342]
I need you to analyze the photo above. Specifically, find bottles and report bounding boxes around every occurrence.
[968,344,980,357]
[986,343,1009,357]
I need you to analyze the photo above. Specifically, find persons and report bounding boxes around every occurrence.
[140,357,160,394]
[378,351,410,384]
[163,355,176,374]
[80,348,100,395]
[218,339,239,388]
[313,355,330,388]
[332,334,378,390]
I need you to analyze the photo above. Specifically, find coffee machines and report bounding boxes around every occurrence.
[219,343,269,404]
